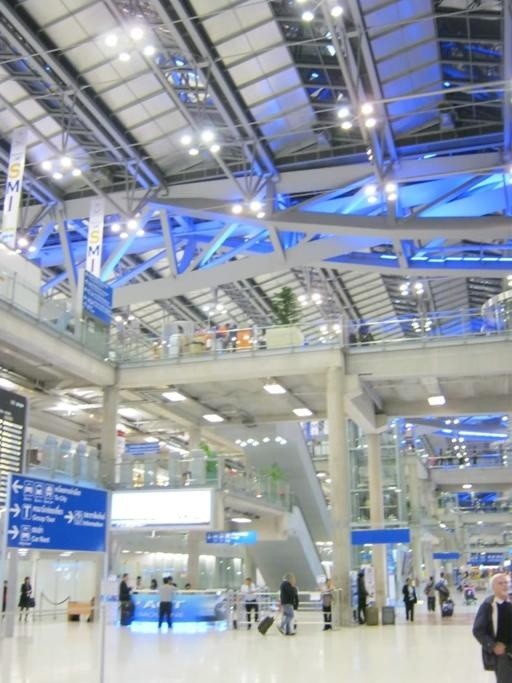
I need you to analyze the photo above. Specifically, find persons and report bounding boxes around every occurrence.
[473,575,512,683]
[119,574,190,630]
[472,500,510,514]
[439,103,462,140]
[353,318,371,346]
[17,576,32,623]
[177,321,266,357]
[1,579,8,618]
[433,447,477,465]
[402,572,476,622]
[241,571,372,636]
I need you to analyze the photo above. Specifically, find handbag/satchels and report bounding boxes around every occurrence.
[434,582,449,596]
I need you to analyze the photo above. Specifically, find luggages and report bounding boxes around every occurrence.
[381,605,395,625]
[440,596,454,617]
[364,606,378,626]
[257,609,281,635]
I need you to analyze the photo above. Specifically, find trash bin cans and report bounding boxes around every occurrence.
[367,607,378,625]
[382,606,395,625]
